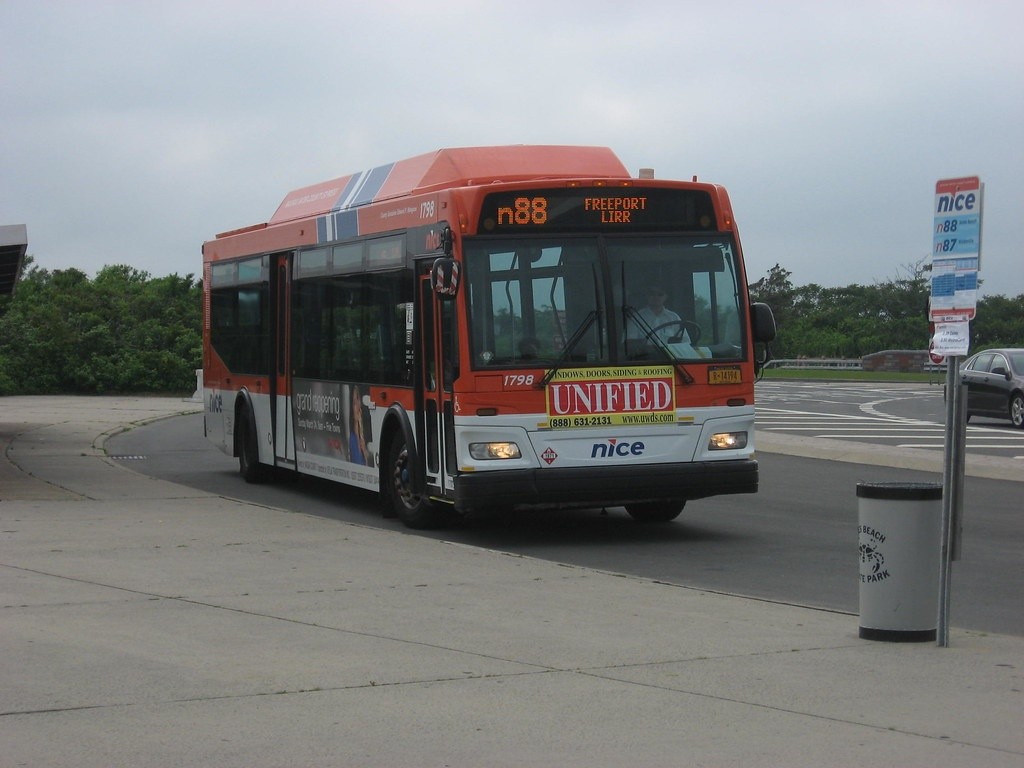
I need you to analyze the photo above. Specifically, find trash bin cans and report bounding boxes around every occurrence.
[851,475,948,646]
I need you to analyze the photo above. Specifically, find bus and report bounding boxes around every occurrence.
[201,144,777,530]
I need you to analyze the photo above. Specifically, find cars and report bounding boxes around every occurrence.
[943,348,1024,428]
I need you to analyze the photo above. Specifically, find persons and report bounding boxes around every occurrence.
[343,386,368,466]
[620,276,692,352]
[513,336,541,364]
